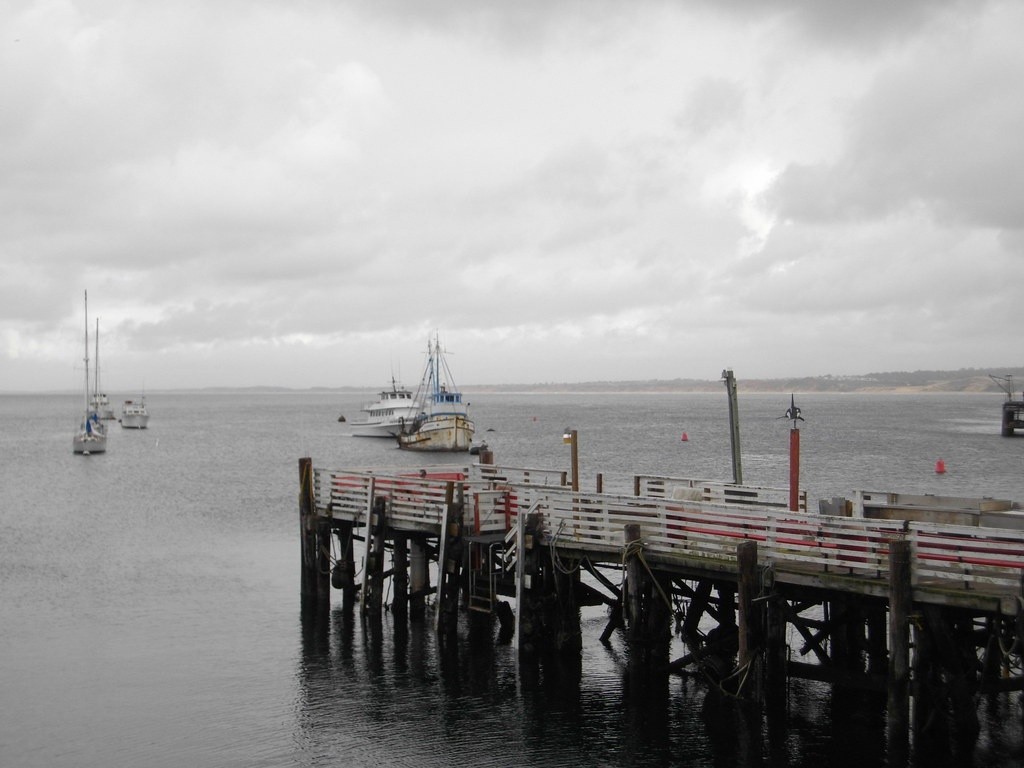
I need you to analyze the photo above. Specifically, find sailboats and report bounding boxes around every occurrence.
[87,317,115,421]
[72,286,107,454]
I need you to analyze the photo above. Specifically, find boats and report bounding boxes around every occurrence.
[120,382,151,429]
[350,372,420,439]
[392,327,475,452]
